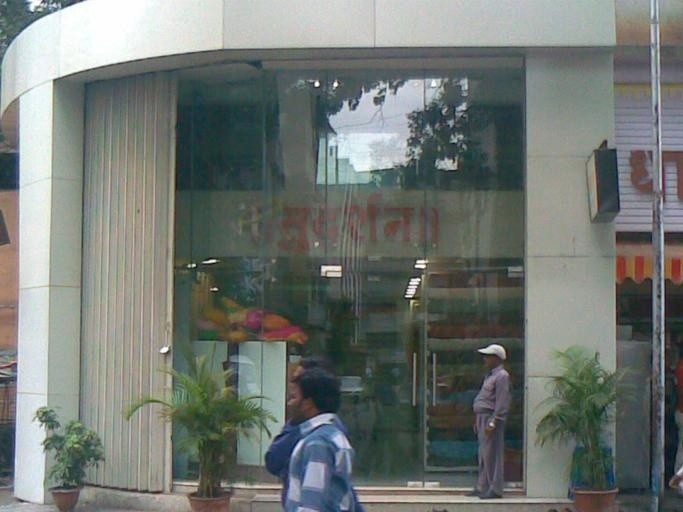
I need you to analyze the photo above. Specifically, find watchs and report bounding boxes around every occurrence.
[488,422,496,428]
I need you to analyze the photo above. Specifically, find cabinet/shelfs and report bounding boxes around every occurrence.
[416,266,526,473]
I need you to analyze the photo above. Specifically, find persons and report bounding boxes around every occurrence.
[673,353,683,498]
[211,355,258,405]
[463,343,512,500]
[667,469,683,490]
[265,358,365,512]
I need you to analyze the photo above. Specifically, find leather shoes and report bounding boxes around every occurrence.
[463,488,503,499]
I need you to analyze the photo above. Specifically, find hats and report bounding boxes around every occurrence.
[477,344,506,361]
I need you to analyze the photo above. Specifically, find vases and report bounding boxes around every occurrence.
[505,446,524,483]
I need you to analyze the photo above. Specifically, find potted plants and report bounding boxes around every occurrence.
[527,341,643,512]
[31,404,108,511]
[120,339,281,512]
